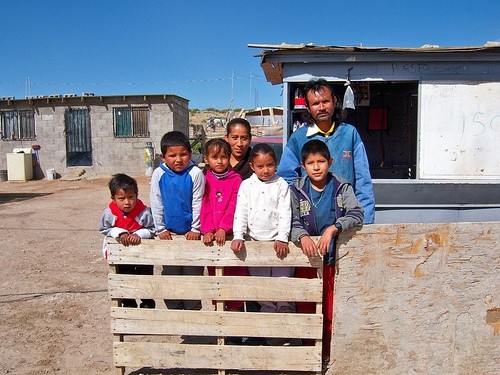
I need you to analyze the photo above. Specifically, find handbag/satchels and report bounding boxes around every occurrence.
[341,80,356,109]
[368,109,387,130]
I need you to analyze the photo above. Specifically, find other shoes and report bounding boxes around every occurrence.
[225,337,267,346]
[321,356,330,372]
[279,306,294,313]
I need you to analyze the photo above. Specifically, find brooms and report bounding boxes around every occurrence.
[31,145,40,181]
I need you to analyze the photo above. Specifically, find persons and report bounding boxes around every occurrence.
[99,174,156,309]
[150,131,207,339]
[199,138,247,311]
[289,139,364,373]
[277,78,374,225]
[204,118,266,345]
[231,143,292,313]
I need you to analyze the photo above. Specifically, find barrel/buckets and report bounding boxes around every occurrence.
[0,170,7,181]
[47,169,56,180]
